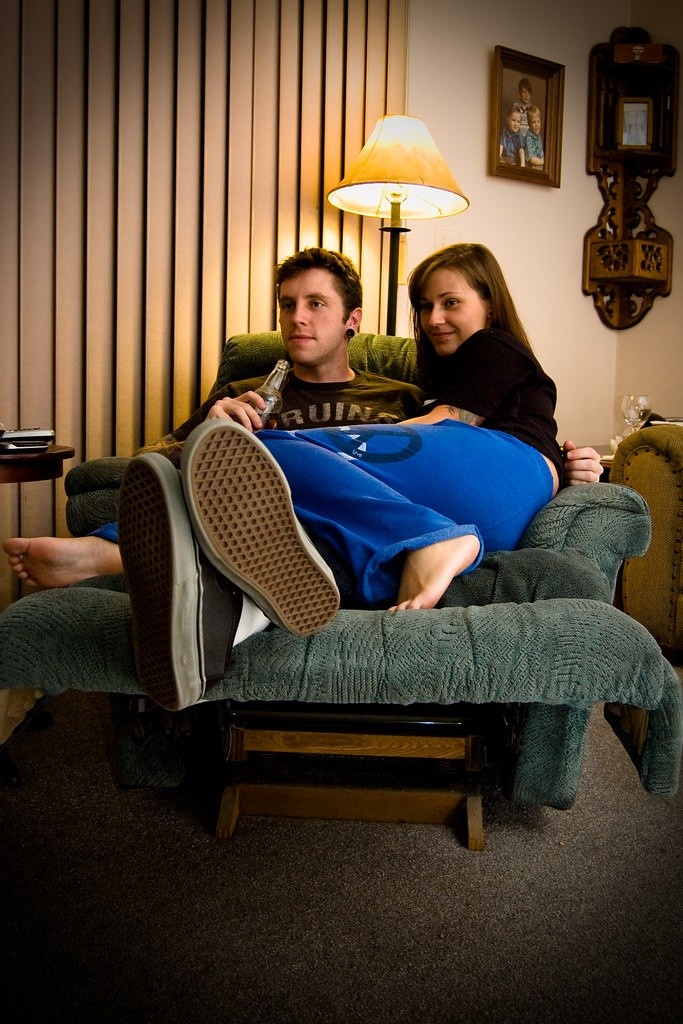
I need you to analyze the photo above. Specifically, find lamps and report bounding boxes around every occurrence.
[326,114,470,336]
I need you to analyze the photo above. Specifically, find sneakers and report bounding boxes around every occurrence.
[117,453,244,711]
[180,418,341,637]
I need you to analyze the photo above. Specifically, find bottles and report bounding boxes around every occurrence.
[247,359,290,429]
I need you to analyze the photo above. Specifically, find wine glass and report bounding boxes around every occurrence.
[622,395,652,432]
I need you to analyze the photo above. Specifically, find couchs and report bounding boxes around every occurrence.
[0,332,682,848]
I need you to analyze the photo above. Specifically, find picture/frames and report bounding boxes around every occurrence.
[486,45,567,189]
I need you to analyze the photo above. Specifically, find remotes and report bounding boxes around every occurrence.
[1,426,56,441]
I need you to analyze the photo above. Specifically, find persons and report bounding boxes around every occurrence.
[118,249,604,710]
[4,245,562,612]
[499,78,545,169]
